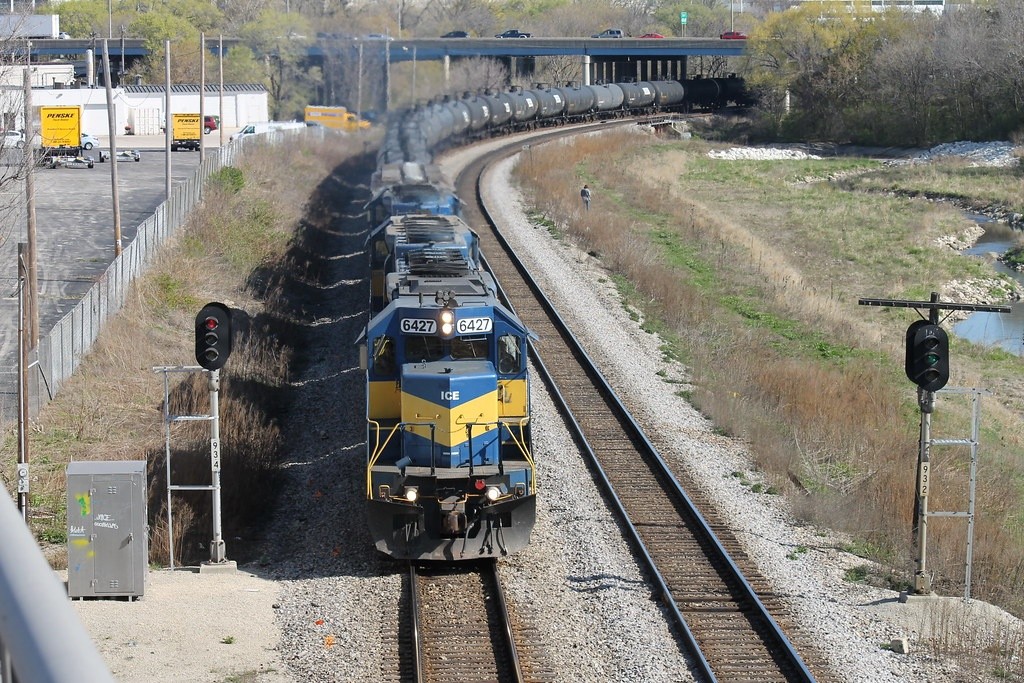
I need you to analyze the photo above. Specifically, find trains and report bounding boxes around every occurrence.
[363,69,766,573]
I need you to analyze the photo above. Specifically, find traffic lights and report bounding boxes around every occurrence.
[914,324,949,391]
[196,306,229,371]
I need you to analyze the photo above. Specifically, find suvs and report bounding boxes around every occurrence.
[204,114,218,135]
[591,28,625,38]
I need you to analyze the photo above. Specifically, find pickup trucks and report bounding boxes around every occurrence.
[494,28,535,39]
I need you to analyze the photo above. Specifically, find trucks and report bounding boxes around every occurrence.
[40,105,82,158]
[172,112,201,152]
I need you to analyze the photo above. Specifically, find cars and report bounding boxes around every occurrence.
[0,130,27,150]
[440,30,471,38]
[276,31,394,42]
[720,31,748,39]
[635,32,665,38]
[82,132,100,151]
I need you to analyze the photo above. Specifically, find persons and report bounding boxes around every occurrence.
[498,339,507,358]
[581,184,590,210]
